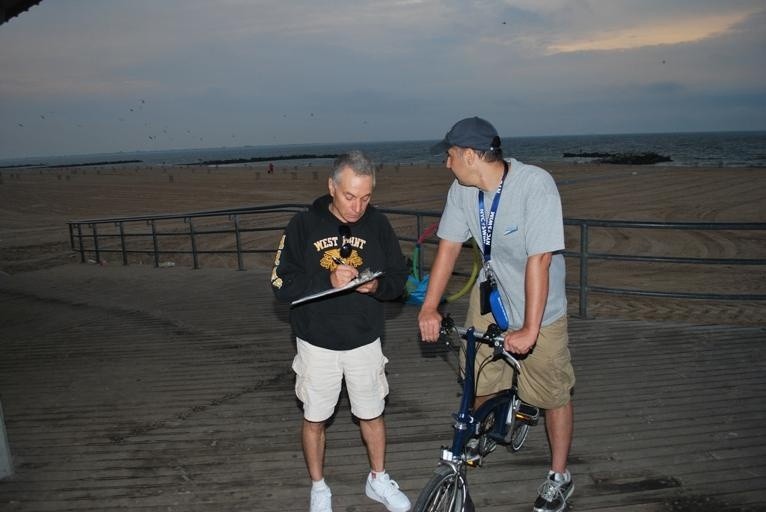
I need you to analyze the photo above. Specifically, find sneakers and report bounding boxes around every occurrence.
[532,467,574,512]
[365,472,411,512]
[309,480,333,512]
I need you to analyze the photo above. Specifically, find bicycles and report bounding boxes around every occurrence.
[411,312,540,512]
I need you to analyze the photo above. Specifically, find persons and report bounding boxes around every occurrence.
[271,148,411,512]
[418,116,574,512]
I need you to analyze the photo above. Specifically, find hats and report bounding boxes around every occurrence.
[430,117,502,157]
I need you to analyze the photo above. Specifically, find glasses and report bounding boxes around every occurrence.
[339,223,352,258]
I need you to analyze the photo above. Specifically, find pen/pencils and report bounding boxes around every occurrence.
[331,255,359,283]
